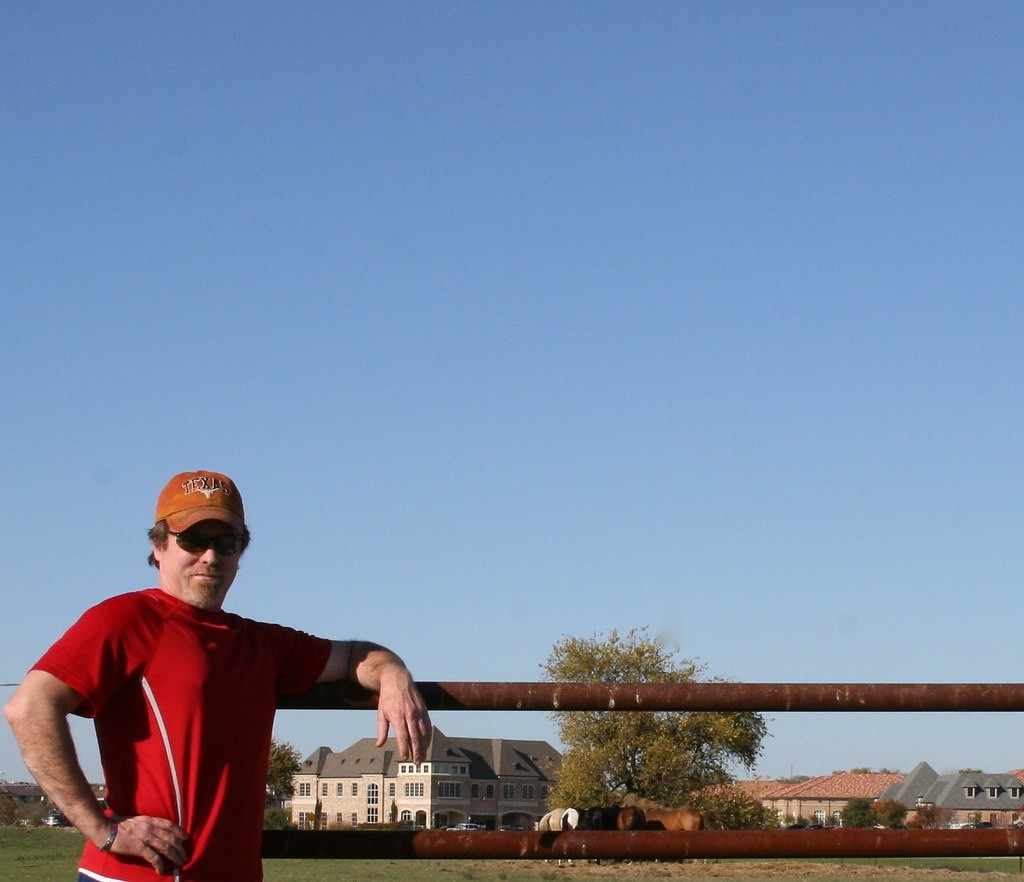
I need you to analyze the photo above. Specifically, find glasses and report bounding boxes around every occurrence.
[167,529,243,556]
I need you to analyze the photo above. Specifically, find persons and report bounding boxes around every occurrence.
[3,470,432,882]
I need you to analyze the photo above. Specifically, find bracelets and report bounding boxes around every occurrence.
[98,820,118,852]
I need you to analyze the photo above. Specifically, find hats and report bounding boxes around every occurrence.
[155,470,244,536]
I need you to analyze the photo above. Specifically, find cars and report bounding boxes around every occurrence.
[944,821,994,830]
[417,822,531,831]
[783,823,833,829]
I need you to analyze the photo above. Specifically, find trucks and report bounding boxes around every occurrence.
[47,808,70,827]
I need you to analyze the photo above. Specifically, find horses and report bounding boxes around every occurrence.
[535,806,706,867]
